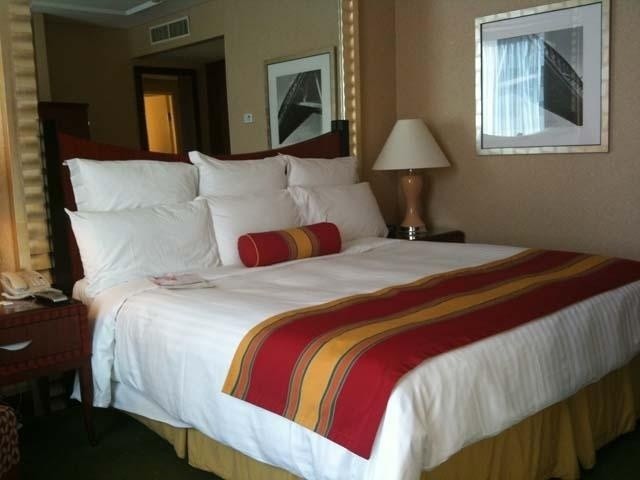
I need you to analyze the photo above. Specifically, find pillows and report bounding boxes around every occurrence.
[187,145,287,194]
[280,151,359,187]
[292,180,391,241]
[66,198,224,277]
[236,219,343,267]
[196,185,301,268]
[62,149,199,200]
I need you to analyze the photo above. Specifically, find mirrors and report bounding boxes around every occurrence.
[1,1,367,278]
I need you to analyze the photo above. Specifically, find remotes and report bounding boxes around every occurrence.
[34,290,69,305]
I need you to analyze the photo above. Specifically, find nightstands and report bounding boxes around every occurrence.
[392,228,466,246]
[1,297,95,444]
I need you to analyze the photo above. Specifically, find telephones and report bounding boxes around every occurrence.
[0,270,51,300]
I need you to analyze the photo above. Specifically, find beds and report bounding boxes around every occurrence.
[48,126,640,479]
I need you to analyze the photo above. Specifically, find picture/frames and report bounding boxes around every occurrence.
[264,48,340,149]
[469,1,615,157]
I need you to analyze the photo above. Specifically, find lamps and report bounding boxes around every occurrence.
[373,116,455,232]
